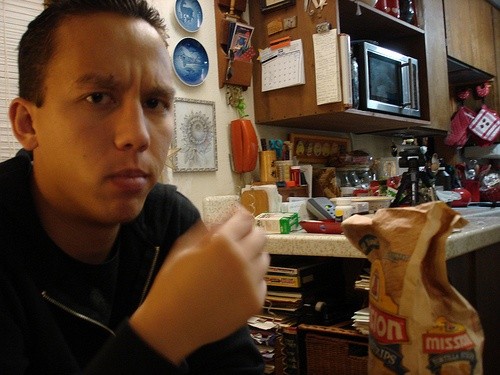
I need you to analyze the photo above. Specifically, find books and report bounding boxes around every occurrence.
[351,305,371,335]
[248,258,321,375]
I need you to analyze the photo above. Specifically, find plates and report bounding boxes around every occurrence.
[172,36,210,87]
[174,0,204,33]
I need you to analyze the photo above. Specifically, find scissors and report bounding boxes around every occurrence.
[269,139,283,161]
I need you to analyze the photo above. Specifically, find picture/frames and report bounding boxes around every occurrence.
[172,96,219,174]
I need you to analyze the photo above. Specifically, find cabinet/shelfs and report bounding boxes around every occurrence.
[250,0,500,138]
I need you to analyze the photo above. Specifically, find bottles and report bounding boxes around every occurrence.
[290,165,301,186]
[434,167,451,201]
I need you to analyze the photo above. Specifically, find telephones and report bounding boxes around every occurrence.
[231,119,258,174]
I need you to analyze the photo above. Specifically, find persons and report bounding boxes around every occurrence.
[0,0,270,375]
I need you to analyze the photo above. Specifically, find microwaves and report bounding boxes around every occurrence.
[351,39,422,119]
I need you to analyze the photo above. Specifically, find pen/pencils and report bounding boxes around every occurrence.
[282,143,289,160]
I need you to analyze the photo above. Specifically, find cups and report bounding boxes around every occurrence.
[275,160,292,183]
[259,150,277,184]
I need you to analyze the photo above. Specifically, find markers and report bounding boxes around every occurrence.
[261,138,267,151]
